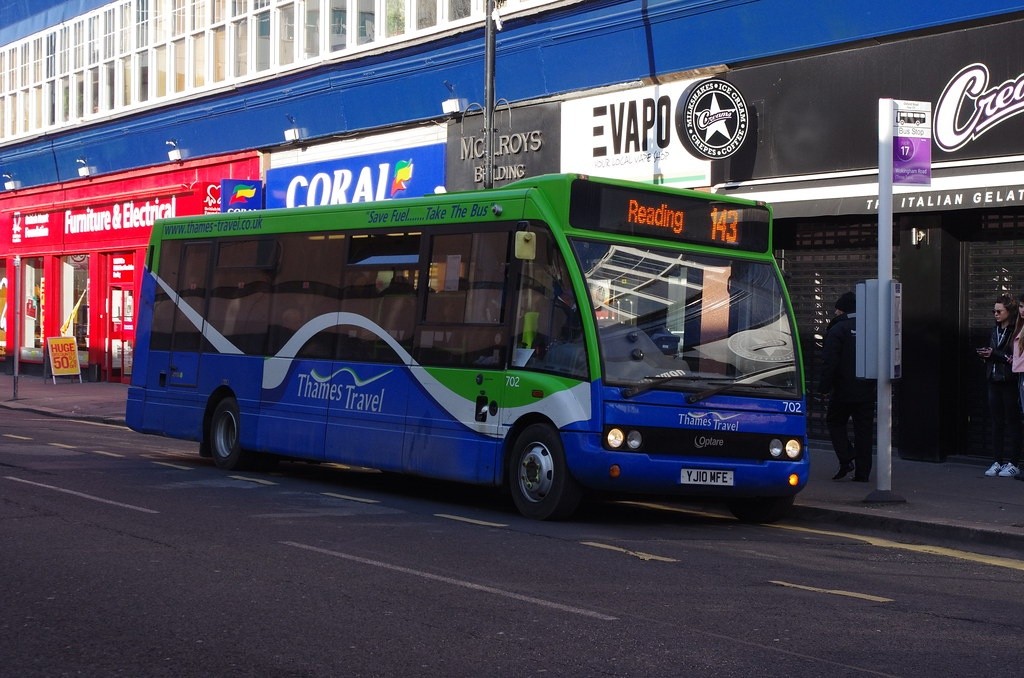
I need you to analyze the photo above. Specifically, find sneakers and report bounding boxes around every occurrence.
[984,462,1021,477]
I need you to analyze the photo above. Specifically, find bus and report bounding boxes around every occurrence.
[126,174,811,522]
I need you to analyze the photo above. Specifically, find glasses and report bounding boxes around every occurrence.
[992,309,1007,314]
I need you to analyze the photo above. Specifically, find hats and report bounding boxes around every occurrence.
[834,292,856,313]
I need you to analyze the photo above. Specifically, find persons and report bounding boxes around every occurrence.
[977,293,1024,481]
[559,283,616,342]
[817,293,876,482]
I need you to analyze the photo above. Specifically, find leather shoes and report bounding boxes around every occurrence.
[832,462,854,480]
[852,474,869,482]
